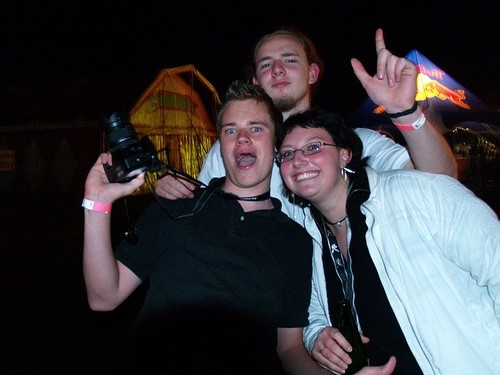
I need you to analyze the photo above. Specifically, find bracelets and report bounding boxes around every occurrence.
[82,197,113,213]
[392,110,428,131]
[385,100,418,118]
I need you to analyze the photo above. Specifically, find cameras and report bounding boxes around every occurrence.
[102,113,160,181]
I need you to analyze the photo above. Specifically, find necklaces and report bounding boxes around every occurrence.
[326,216,347,227]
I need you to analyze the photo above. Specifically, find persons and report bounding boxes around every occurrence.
[83,83,396,375]
[197,28,458,227]
[275,111,500,375]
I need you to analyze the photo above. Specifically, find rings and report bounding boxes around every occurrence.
[319,345,325,352]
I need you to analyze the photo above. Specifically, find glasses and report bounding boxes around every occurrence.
[275,141,336,164]
